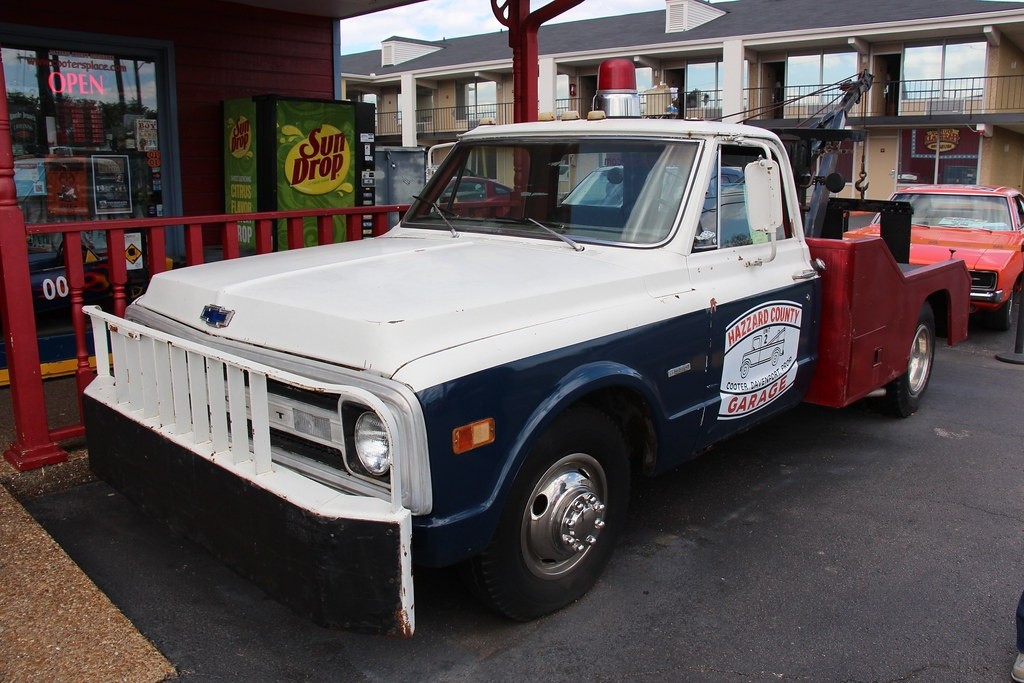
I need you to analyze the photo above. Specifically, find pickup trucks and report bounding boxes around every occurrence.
[80,104,974,633]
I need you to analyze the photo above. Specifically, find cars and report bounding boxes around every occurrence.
[27,234,145,319]
[457,167,478,176]
[432,176,518,218]
[558,165,744,235]
[862,183,1024,327]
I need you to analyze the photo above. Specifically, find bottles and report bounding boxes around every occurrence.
[32,94,59,158]
[125,131,136,151]
